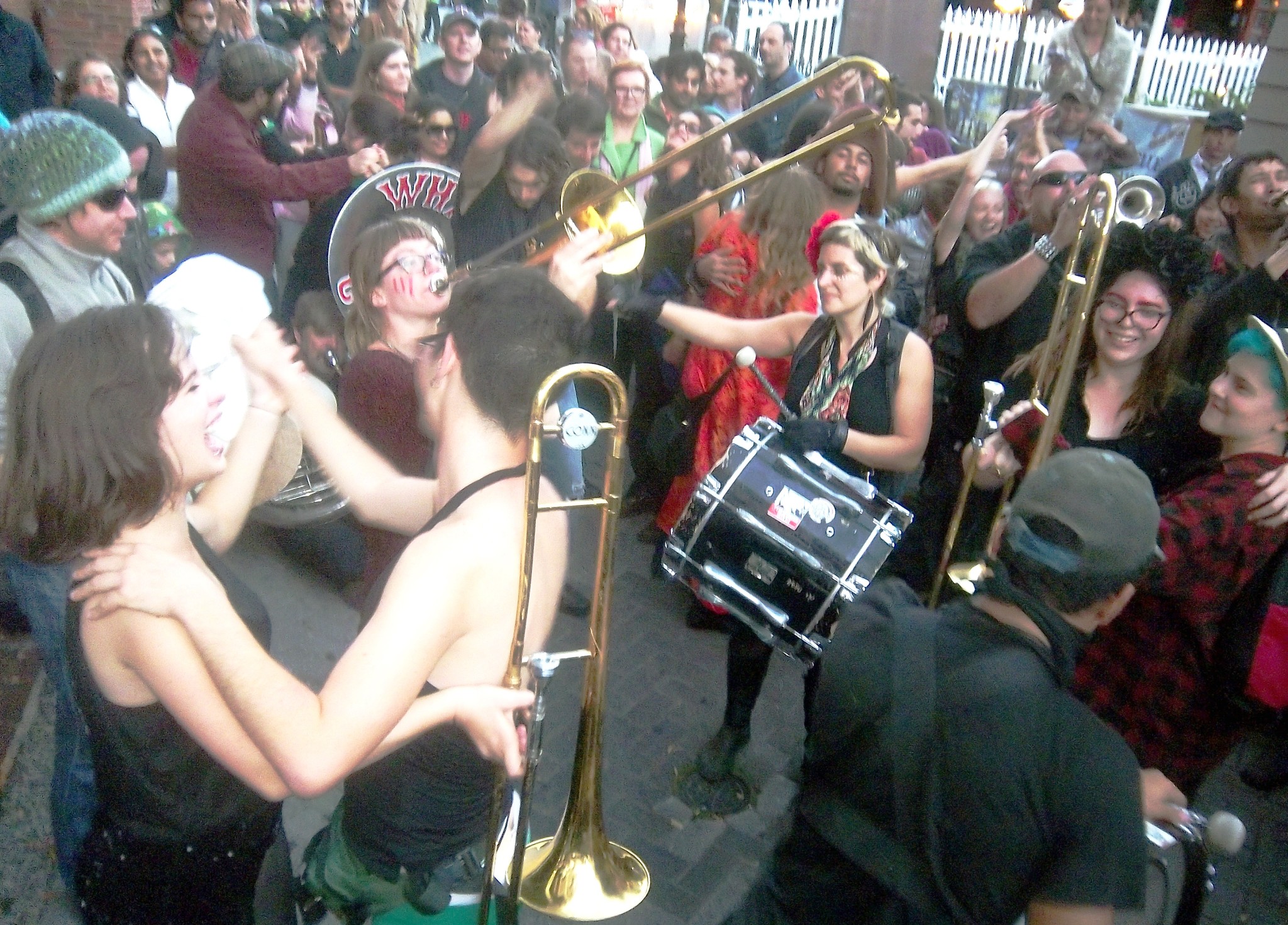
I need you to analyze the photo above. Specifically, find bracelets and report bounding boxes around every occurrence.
[1034,234,1059,262]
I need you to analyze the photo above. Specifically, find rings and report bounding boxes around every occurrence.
[1069,197,1076,205]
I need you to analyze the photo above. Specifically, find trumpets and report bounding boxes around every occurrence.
[1096,176,1167,229]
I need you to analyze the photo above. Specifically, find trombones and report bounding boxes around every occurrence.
[928,173,1114,605]
[433,55,891,289]
[480,360,652,921]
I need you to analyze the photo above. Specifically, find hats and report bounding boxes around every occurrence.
[1206,109,1245,130]
[223,40,296,86]
[440,10,481,34]
[0,110,132,223]
[1245,315,1287,387]
[1060,88,1091,106]
[1005,449,1165,576]
[806,105,889,218]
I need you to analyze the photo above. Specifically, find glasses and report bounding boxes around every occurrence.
[372,246,450,283]
[1030,172,1087,186]
[423,125,456,138]
[414,331,465,358]
[612,85,644,97]
[671,119,701,136]
[1095,300,1171,331]
[91,188,128,213]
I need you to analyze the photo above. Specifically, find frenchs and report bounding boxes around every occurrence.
[248,161,461,529]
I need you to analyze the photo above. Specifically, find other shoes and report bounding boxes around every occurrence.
[698,722,751,781]
[1242,733,1287,793]
[636,523,663,543]
[558,582,590,620]
[617,482,664,516]
[339,581,367,611]
[685,603,735,633]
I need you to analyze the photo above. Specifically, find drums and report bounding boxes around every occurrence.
[1140,805,1212,923]
[663,416,914,668]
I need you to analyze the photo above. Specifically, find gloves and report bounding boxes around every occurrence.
[779,420,850,455]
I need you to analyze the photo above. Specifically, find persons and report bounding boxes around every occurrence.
[177,40,387,329]
[1150,109,1241,236]
[453,59,898,630]
[1,301,537,923]
[959,237,1288,531]
[1,109,137,892]
[604,219,934,781]
[1000,0,1136,234]
[893,109,1032,608]
[1165,152,1288,382]
[937,149,1114,564]
[716,447,1184,925]
[339,216,612,624]
[149,1,966,234]
[69,262,568,925]
[256,97,414,323]
[1069,314,1288,798]
[0,7,192,301]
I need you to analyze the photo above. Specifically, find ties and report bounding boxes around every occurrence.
[1202,162,1222,196]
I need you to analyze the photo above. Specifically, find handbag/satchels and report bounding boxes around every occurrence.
[646,388,712,474]
